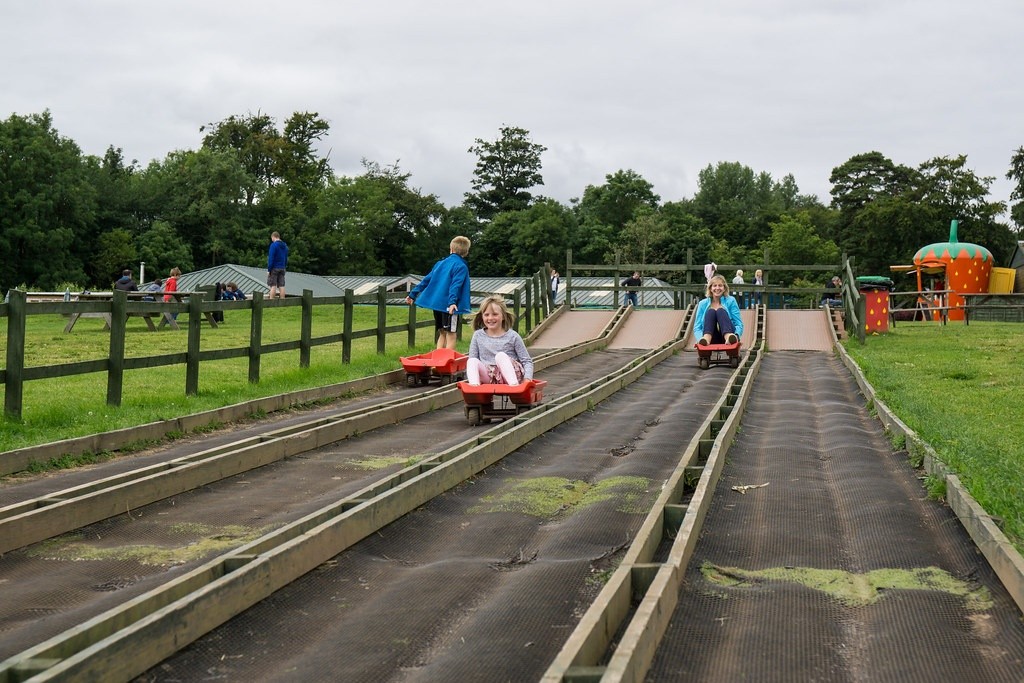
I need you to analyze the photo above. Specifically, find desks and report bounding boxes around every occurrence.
[63,291,219,333]
[889,290,955,328]
[957,293,1024,327]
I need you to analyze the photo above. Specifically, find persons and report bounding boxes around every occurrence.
[405,236,472,351]
[621,272,641,309]
[266,231,289,300]
[732,269,744,309]
[550,267,560,306]
[693,275,744,346]
[465,294,534,386]
[749,269,763,306]
[114,267,247,320]
[821,276,843,308]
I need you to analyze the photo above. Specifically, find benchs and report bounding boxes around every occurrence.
[889,305,1024,328]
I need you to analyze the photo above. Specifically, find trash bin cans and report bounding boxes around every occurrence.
[857,275,893,333]
